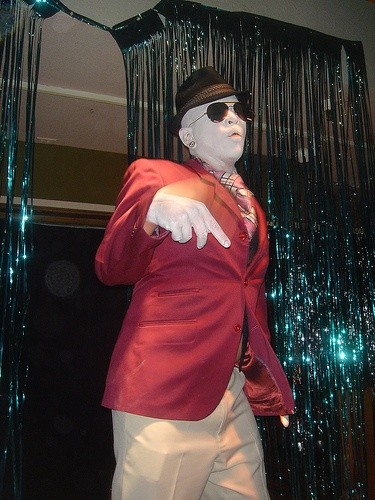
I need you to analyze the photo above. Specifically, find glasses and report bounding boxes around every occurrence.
[189,101,253,126]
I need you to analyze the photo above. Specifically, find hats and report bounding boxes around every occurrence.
[167,66,250,136]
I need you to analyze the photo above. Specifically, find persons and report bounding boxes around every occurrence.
[95,66,295,500]
